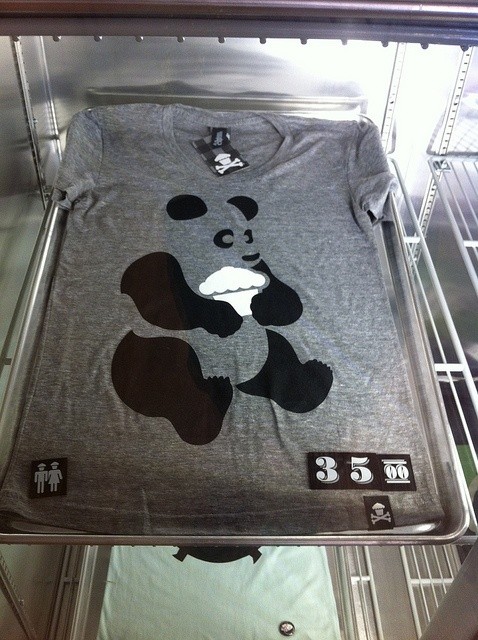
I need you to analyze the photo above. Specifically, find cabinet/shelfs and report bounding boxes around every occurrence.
[0,1,478,640]
